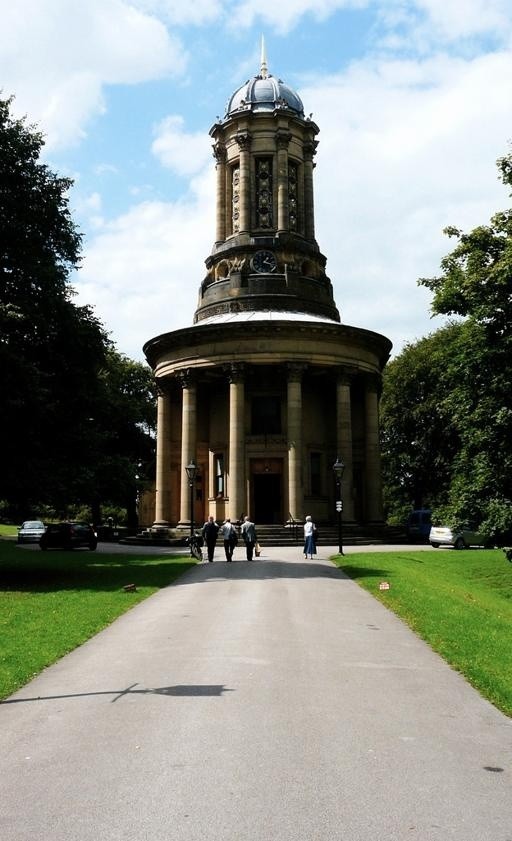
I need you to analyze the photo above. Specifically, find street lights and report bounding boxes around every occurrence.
[185,459,198,556]
[333,459,345,555]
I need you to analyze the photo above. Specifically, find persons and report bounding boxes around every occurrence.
[302,515,317,560]
[240,516,257,562]
[221,518,237,562]
[201,516,219,562]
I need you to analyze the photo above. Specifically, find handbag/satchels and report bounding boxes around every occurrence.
[255,542,261,557]
[312,523,318,543]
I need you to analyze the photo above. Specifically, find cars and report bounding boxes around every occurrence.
[404,510,435,540]
[40,523,98,551]
[17,520,48,543]
[429,520,495,549]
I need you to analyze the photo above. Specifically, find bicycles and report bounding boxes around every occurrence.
[187,532,202,560]
